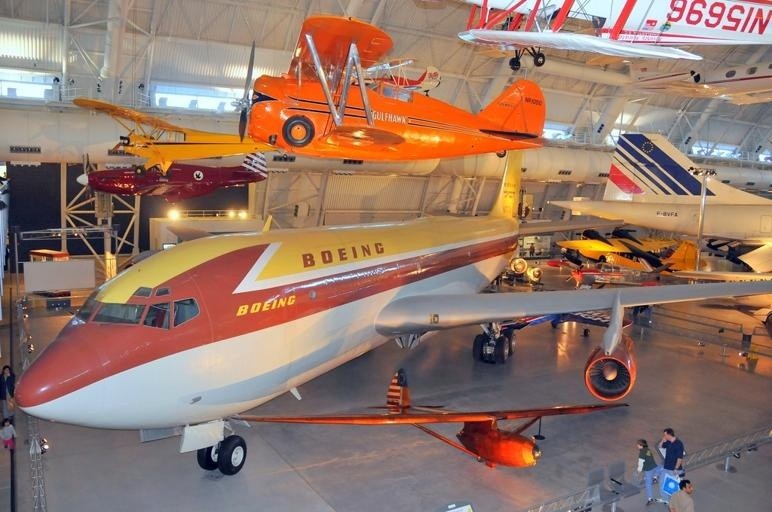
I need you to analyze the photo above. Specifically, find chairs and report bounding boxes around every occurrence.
[607,461,640,498]
[586,468,620,505]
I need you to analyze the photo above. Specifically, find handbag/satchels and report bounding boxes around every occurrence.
[7,398,15,411]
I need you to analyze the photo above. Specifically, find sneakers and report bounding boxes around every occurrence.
[646,499,653,506]
[655,498,664,502]
[664,501,669,504]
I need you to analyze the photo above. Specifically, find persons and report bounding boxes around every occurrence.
[658,428,685,504]
[0,365,16,424]
[636,439,661,506]
[668,480,694,512]
[528,243,535,257]
[0,418,18,451]
